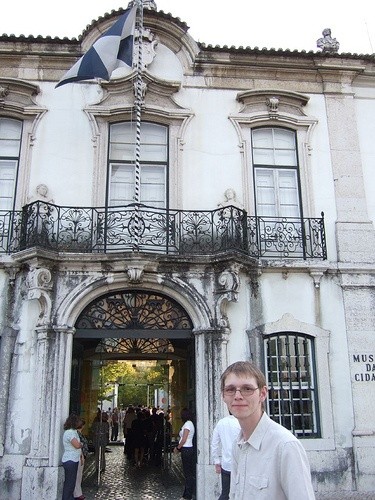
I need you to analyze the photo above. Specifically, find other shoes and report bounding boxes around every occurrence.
[75,495,86,500]
[180,497,193,500]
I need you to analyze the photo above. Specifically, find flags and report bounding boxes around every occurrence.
[54,6,137,90]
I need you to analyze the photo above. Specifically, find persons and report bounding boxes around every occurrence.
[121,409,126,419]
[92,412,110,473]
[218,189,243,245]
[122,404,173,471]
[62,414,84,500]
[29,184,55,245]
[174,409,195,500]
[73,416,88,500]
[97,408,101,420]
[219,361,316,500]
[106,407,121,441]
[317,27,340,53]
[211,406,241,500]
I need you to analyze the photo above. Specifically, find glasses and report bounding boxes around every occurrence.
[221,384,263,396]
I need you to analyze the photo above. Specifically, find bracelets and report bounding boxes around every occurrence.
[176,446,180,450]
